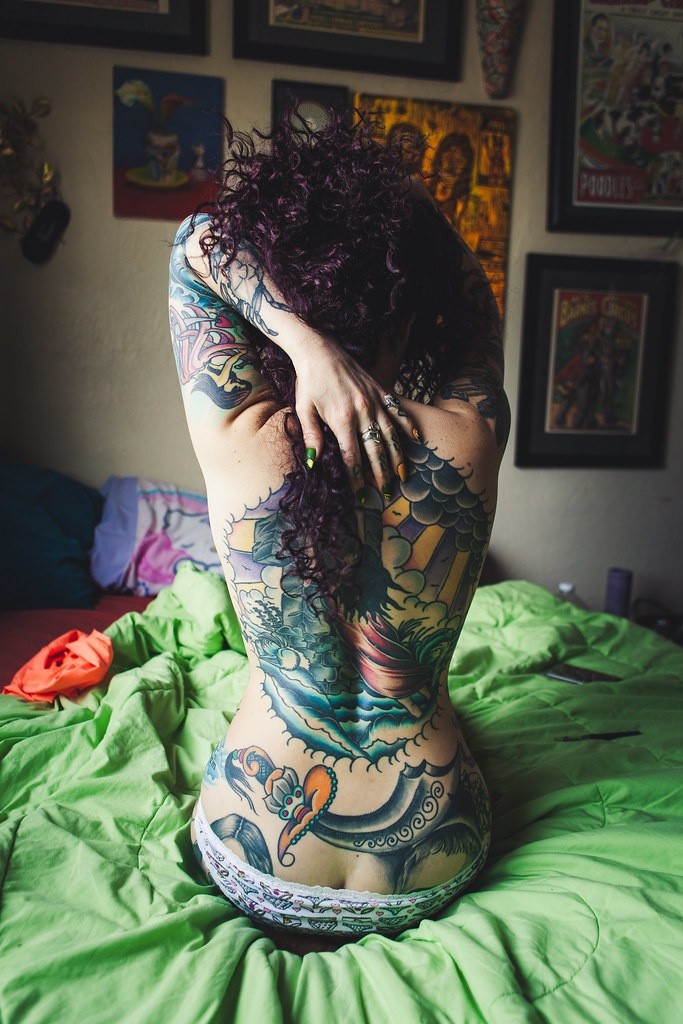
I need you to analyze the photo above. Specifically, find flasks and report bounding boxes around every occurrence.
[602,565,631,616]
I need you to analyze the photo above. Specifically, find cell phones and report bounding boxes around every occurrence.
[542,662,623,686]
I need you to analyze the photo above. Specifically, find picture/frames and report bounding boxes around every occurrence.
[352,92,516,324]
[112,64,225,223]
[545,0,682,239]
[231,0,466,84]
[514,250,683,470]
[1,1,213,58]
[270,78,350,153]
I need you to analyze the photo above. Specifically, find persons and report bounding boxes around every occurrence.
[166,93,510,935]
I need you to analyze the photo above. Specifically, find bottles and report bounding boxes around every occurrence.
[555,581,574,603]
